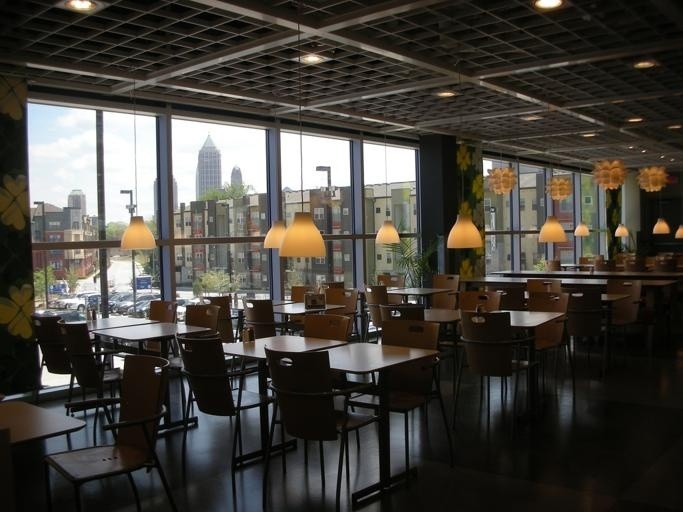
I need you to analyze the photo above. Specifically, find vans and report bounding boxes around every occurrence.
[133,275,151,289]
[52,283,67,293]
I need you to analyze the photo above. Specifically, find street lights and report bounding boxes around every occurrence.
[316,164,333,283]
[32,199,49,309]
[220,203,233,293]
[118,188,137,317]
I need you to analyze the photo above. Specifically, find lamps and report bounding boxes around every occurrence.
[572,146,591,237]
[674,223,682,240]
[277,1,329,258]
[652,152,671,235]
[119,2,157,252]
[263,43,288,249]
[374,92,401,245]
[536,56,569,245]
[445,56,483,250]
[614,182,628,238]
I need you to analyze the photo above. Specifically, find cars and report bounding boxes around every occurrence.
[32,291,203,322]
[48,281,67,292]
